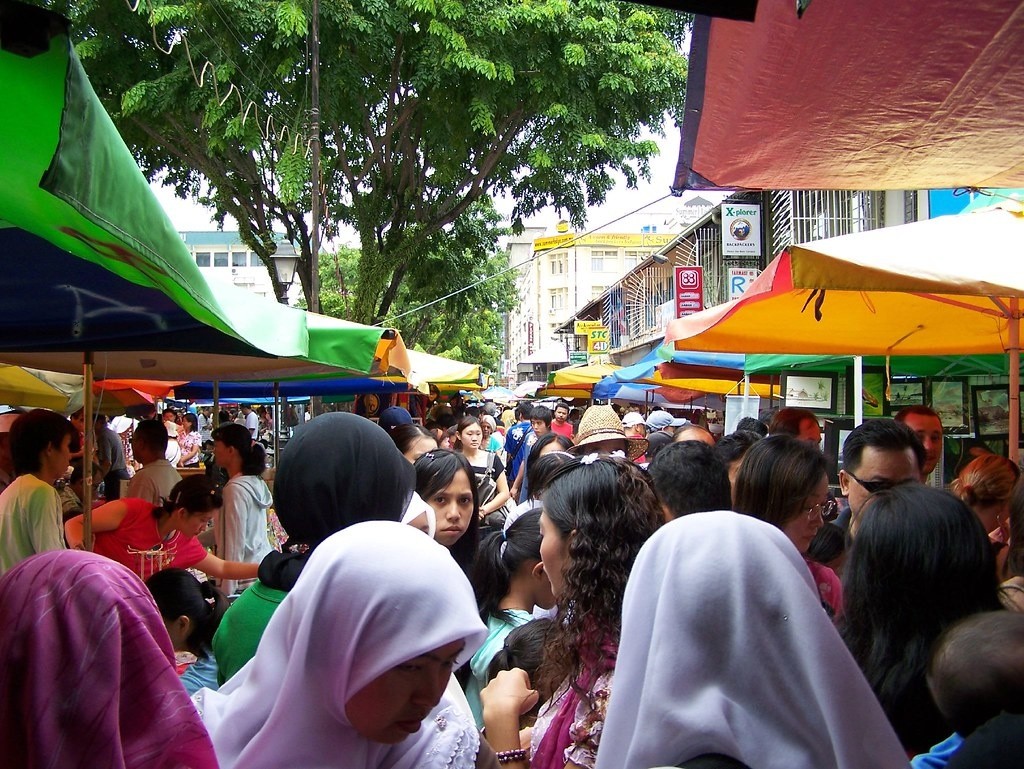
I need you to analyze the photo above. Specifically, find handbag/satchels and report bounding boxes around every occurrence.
[475,453,509,529]
[504,457,513,476]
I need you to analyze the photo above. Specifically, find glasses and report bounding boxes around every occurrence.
[844,469,918,494]
[92,483,99,490]
[801,500,836,521]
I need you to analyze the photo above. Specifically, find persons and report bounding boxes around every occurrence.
[1,399,664,768]
[627,399,1024,768]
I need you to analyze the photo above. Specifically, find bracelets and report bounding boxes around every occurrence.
[497,749,528,764]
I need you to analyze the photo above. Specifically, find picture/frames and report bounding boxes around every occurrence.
[780,369,837,414]
[926,375,969,434]
[890,378,926,410]
[1018,385,1024,441]
[845,365,891,418]
[972,384,1010,441]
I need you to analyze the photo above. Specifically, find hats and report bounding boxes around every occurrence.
[378,406,413,430]
[164,420,178,438]
[565,404,649,461]
[622,412,647,428]
[646,410,686,432]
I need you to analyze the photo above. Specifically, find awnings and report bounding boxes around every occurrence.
[479,1,1023,429]
[0,0,490,552]
